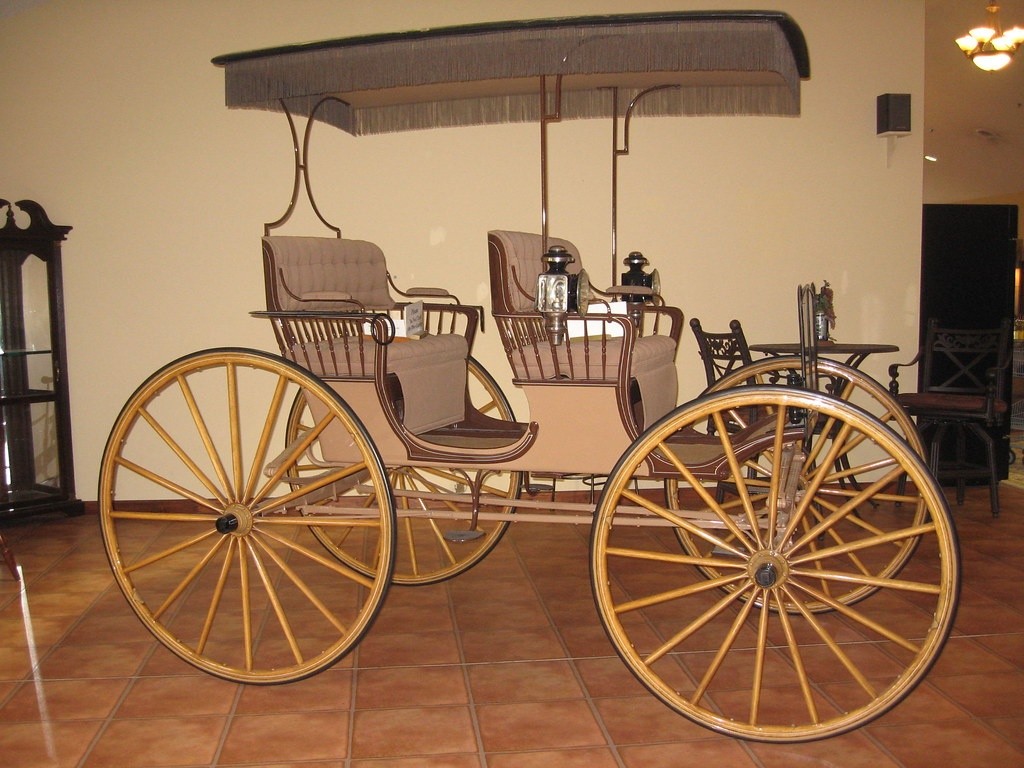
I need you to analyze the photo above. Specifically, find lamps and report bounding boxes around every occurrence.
[956,0,1024,71]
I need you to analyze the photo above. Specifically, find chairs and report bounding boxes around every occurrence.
[690,317,826,543]
[889,318,1011,516]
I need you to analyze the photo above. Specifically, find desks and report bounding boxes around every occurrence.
[749,343,899,517]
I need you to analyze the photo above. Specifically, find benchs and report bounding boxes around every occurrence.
[488,229,684,432]
[262,235,479,435]
